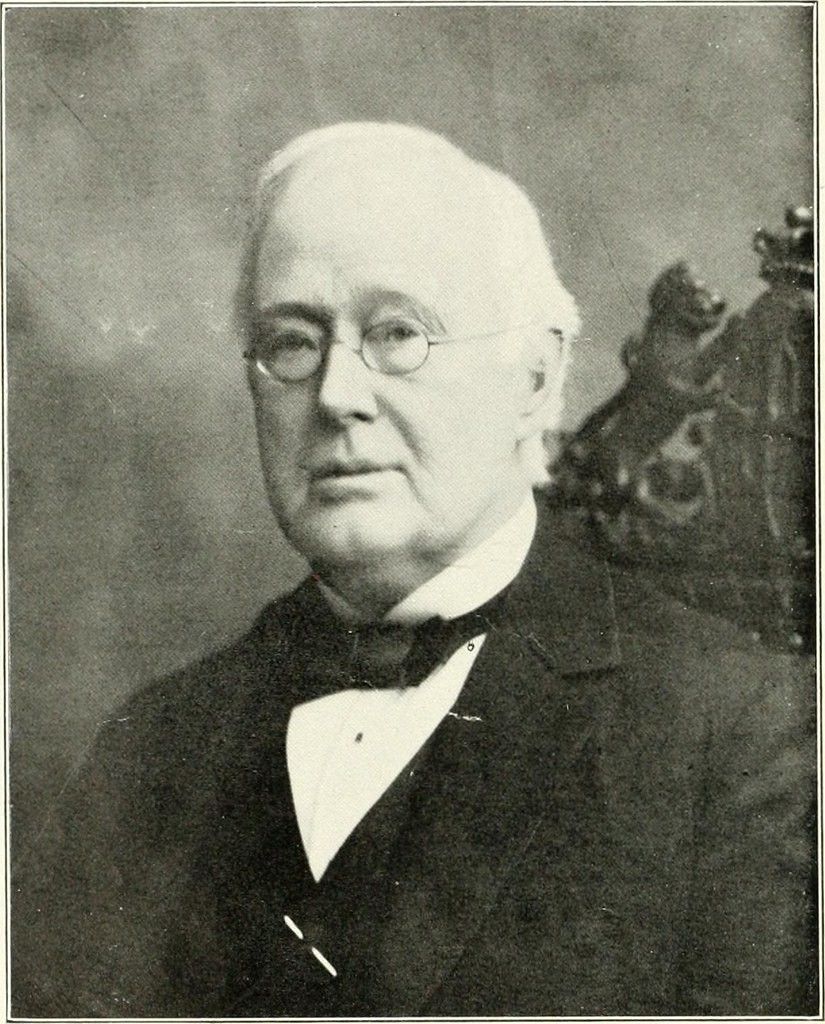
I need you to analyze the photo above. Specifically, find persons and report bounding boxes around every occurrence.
[17,115,820,1013]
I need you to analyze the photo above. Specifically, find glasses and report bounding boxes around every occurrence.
[242,319,526,385]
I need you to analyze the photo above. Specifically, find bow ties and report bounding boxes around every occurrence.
[272,577,514,710]
